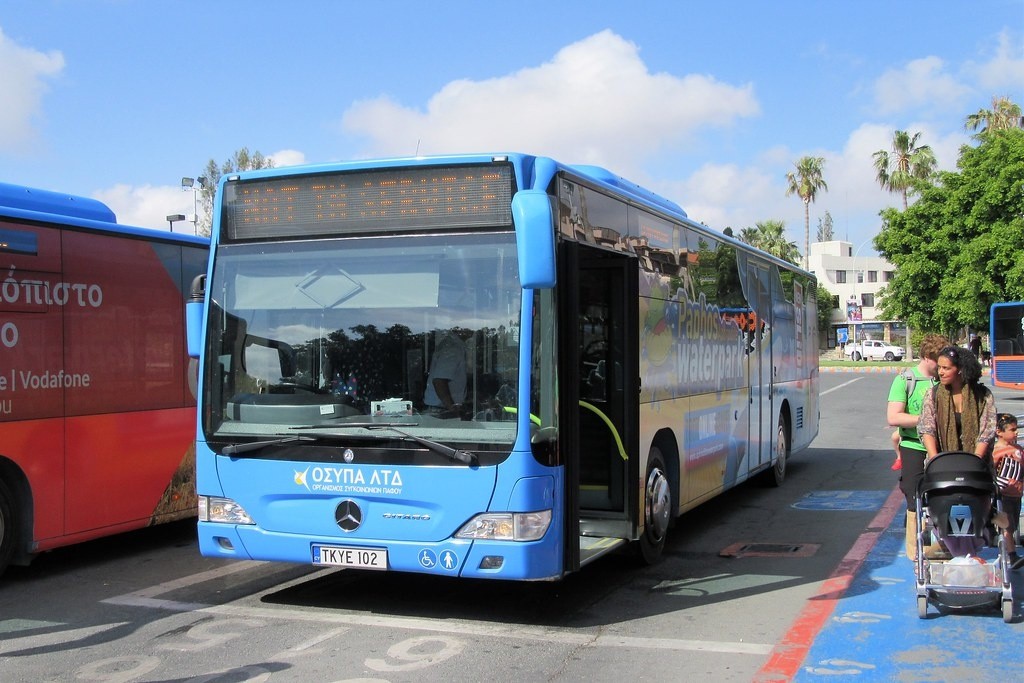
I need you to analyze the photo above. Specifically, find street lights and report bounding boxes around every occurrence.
[166,212,185,235]
[182,176,209,237]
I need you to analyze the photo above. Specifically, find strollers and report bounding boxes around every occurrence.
[912,450,1014,624]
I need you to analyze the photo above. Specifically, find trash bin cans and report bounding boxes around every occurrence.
[867,355,873,362]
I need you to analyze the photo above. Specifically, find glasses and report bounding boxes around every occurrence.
[996,414,1012,427]
[940,348,959,359]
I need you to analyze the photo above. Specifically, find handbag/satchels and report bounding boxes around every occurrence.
[943,553,989,587]
[996,457,1024,491]
[906,508,954,562]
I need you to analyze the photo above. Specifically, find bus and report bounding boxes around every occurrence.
[0,180,214,590]
[989,302,1024,400]
[184,154,821,584]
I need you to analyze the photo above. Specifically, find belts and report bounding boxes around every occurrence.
[424,405,448,410]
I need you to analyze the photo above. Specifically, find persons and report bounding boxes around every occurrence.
[530,371,538,414]
[915,345,997,470]
[886,332,952,530]
[585,359,624,398]
[992,412,1024,571]
[890,429,902,471]
[971,336,991,367]
[423,314,475,418]
[494,376,516,419]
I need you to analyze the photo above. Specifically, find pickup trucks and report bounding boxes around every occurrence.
[843,339,905,362]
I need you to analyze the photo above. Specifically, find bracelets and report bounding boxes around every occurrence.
[973,451,983,458]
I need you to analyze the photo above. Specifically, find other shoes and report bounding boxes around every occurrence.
[1010,556,1024,569]
[891,459,902,471]
